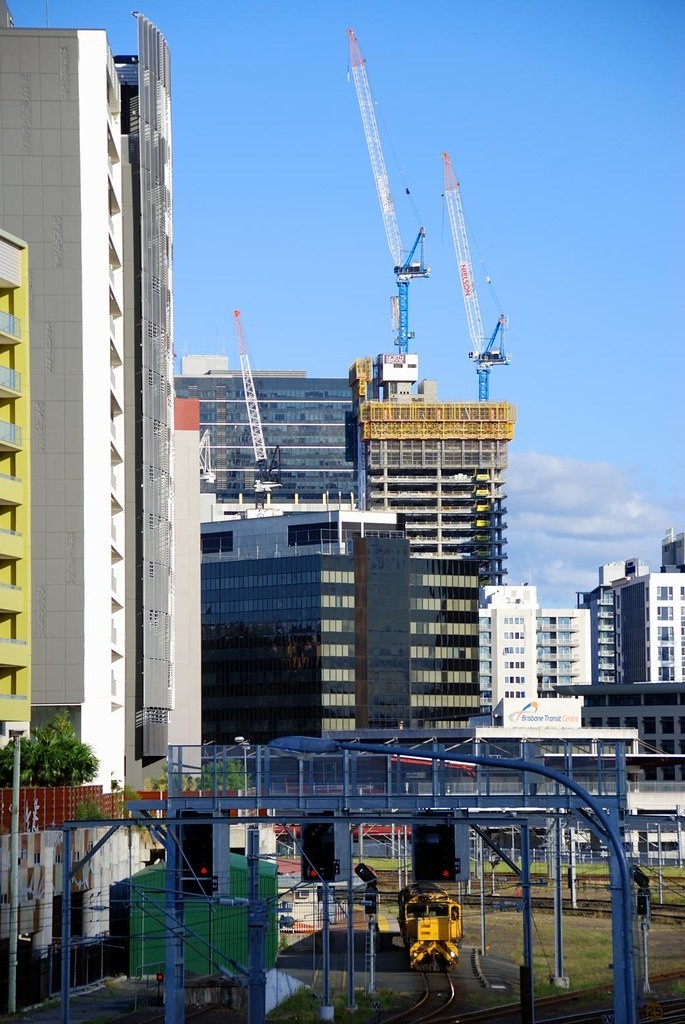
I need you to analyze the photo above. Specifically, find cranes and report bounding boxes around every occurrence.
[345,26,430,358]
[232,309,284,501]
[442,150,511,399]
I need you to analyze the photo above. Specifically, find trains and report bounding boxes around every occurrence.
[396,881,465,972]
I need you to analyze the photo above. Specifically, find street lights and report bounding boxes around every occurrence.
[265,734,639,1023]
